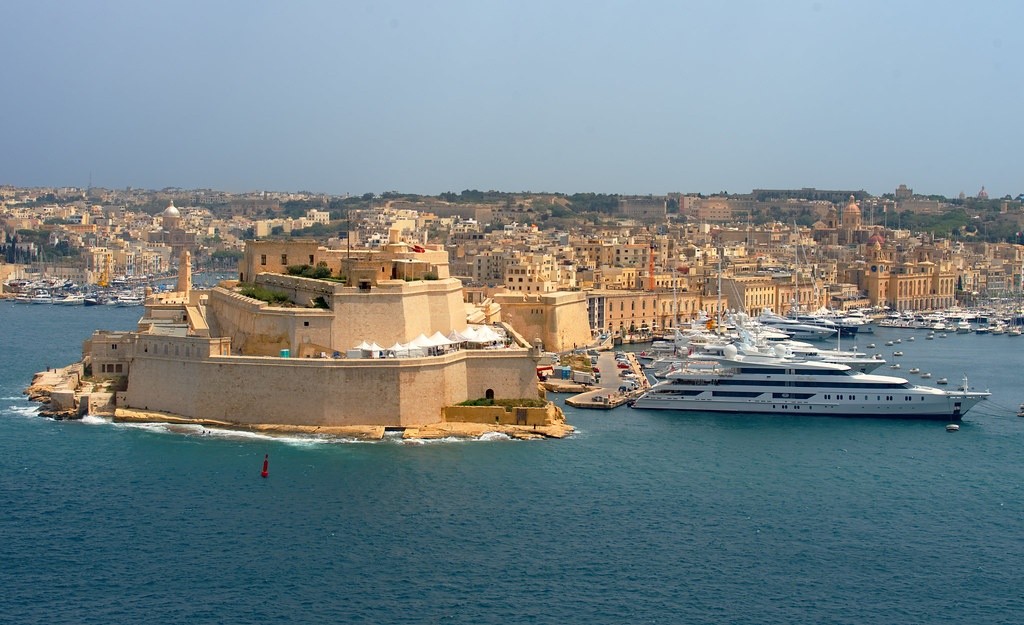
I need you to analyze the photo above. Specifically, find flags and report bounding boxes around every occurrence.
[414,245,425,253]
[406,244,414,250]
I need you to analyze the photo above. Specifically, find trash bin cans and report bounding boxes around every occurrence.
[604,397,609,404]
[279,349,290,358]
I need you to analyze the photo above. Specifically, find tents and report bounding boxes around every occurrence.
[353,324,499,359]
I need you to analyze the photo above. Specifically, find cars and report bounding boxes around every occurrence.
[592,395,608,402]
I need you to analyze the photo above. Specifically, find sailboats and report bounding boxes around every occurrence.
[8,242,241,307]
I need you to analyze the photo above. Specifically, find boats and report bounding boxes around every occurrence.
[588,217,1024,393]
[629,343,994,420]
[946,423,960,431]
